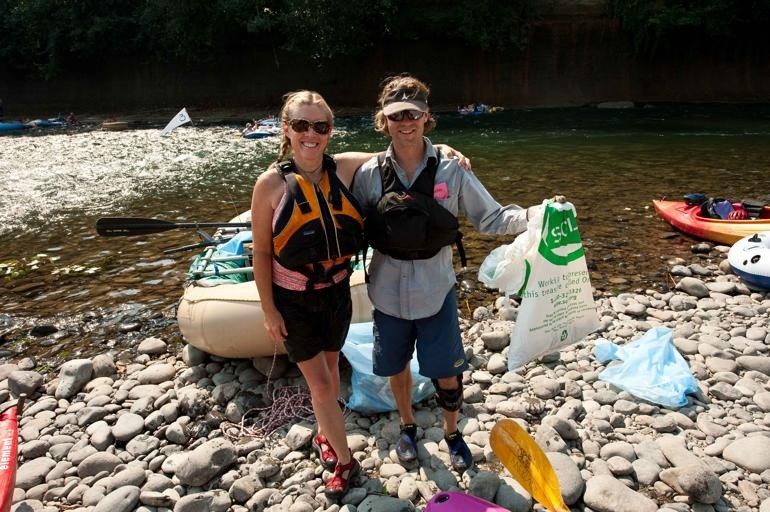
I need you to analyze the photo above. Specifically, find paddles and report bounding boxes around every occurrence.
[96,217,251,236]
[489,420,570,512]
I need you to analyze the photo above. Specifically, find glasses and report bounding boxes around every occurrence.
[286,119,330,135]
[388,111,424,121]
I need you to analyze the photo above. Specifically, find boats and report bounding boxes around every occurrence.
[174,209,383,361]
[458,104,505,117]
[0,115,134,136]
[0,393,28,512]
[243,117,282,139]
[423,490,516,512]
[649,197,770,247]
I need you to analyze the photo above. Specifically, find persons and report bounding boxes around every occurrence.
[250,90,472,499]
[240,121,260,137]
[67,112,77,123]
[58,111,65,120]
[350,74,567,473]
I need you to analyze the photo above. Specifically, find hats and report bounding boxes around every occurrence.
[382,88,429,116]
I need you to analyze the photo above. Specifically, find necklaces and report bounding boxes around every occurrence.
[297,163,322,175]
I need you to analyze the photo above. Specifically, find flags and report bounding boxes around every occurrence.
[159,107,191,137]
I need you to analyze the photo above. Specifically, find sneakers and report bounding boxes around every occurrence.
[396,423,418,462]
[312,433,338,467]
[325,448,360,499]
[444,428,472,472]
